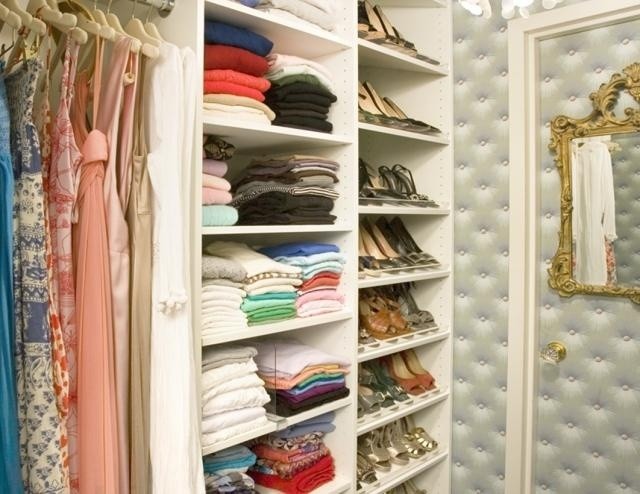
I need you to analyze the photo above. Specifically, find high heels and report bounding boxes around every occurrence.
[359,158,440,208]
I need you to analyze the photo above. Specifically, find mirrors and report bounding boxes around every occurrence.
[547,61,640,305]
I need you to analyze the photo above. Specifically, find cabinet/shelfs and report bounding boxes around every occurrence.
[193,1,452,493]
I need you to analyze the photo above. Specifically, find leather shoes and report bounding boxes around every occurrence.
[358,78,443,135]
[358,0,440,66]
[359,215,439,279]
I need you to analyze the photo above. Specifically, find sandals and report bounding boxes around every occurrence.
[357,282,438,494]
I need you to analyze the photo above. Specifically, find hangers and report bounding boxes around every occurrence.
[0,1,193,69]
